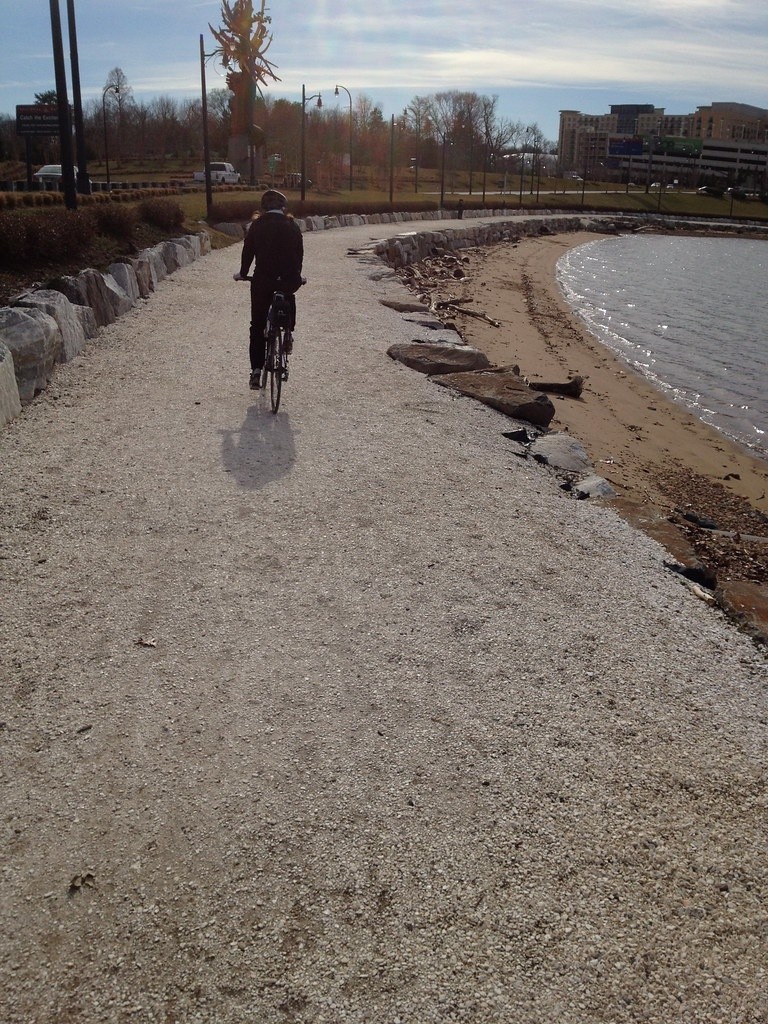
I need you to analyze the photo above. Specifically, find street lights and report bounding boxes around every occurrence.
[461,123,472,195]
[526,158,535,194]
[334,84,353,191]
[581,166,591,204]
[300,83,325,203]
[389,114,406,202]
[102,84,120,190]
[536,163,546,203]
[440,131,454,208]
[482,152,495,202]
[198,32,238,215]
[403,106,419,193]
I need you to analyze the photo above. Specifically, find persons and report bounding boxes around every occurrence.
[234,191,303,386]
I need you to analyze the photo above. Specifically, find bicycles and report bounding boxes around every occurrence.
[234,274,307,415]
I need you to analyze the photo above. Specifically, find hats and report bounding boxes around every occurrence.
[260,190,288,209]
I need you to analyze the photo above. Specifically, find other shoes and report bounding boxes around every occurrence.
[249,373,261,390]
[282,332,293,353]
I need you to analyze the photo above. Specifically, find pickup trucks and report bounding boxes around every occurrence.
[192,161,241,185]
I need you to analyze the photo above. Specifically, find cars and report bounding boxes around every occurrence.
[650,182,661,187]
[32,164,93,183]
[666,184,675,189]
[698,186,707,191]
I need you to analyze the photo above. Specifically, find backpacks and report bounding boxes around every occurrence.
[255,216,301,275]
